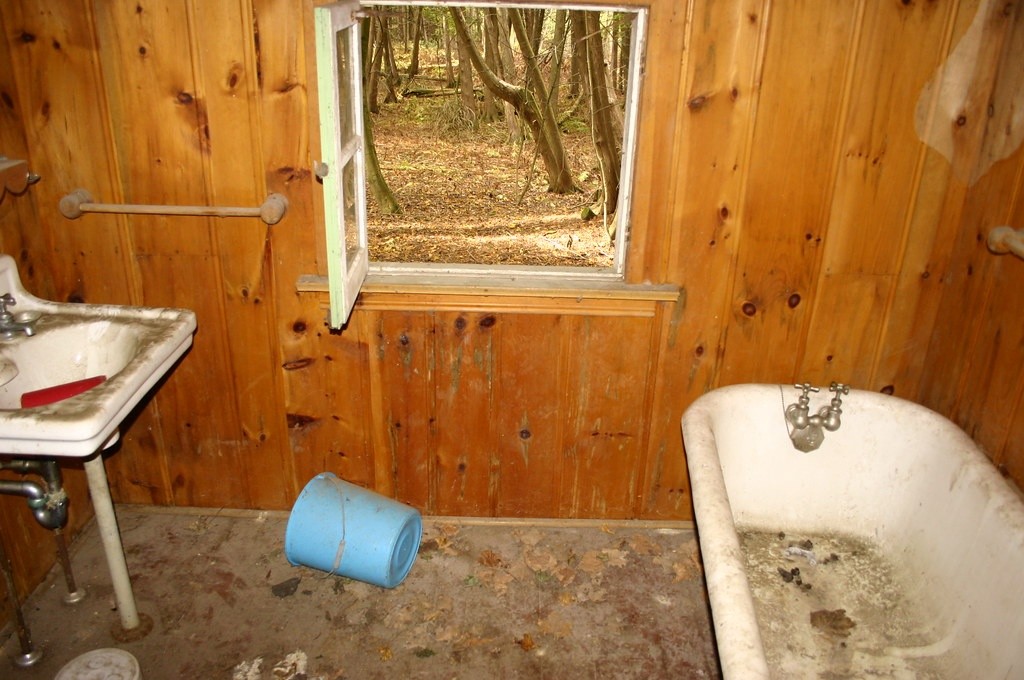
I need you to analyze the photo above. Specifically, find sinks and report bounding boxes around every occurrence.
[1,299,198,456]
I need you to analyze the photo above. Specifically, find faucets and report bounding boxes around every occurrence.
[786,381,850,433]
[0,293,36,341]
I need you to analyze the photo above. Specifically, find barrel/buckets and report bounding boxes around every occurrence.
[285,472,422,588]
[54,648,143,680]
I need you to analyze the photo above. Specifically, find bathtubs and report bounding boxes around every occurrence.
[681,384,1022,680]
